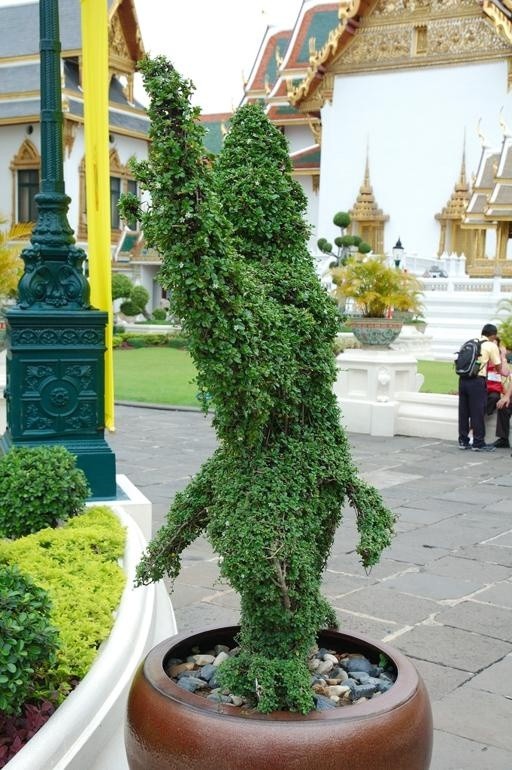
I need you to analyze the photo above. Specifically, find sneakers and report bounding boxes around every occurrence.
[459,439,510,452]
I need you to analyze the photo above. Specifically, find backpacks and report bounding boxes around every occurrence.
[455,338,488,378]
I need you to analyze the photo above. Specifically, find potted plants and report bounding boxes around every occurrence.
[329,250,429,348]
[122,55,436,770]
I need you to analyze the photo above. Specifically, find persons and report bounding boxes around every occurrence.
[485,338,507,416]
[456,324,509,452]
[489,373,512,449]
